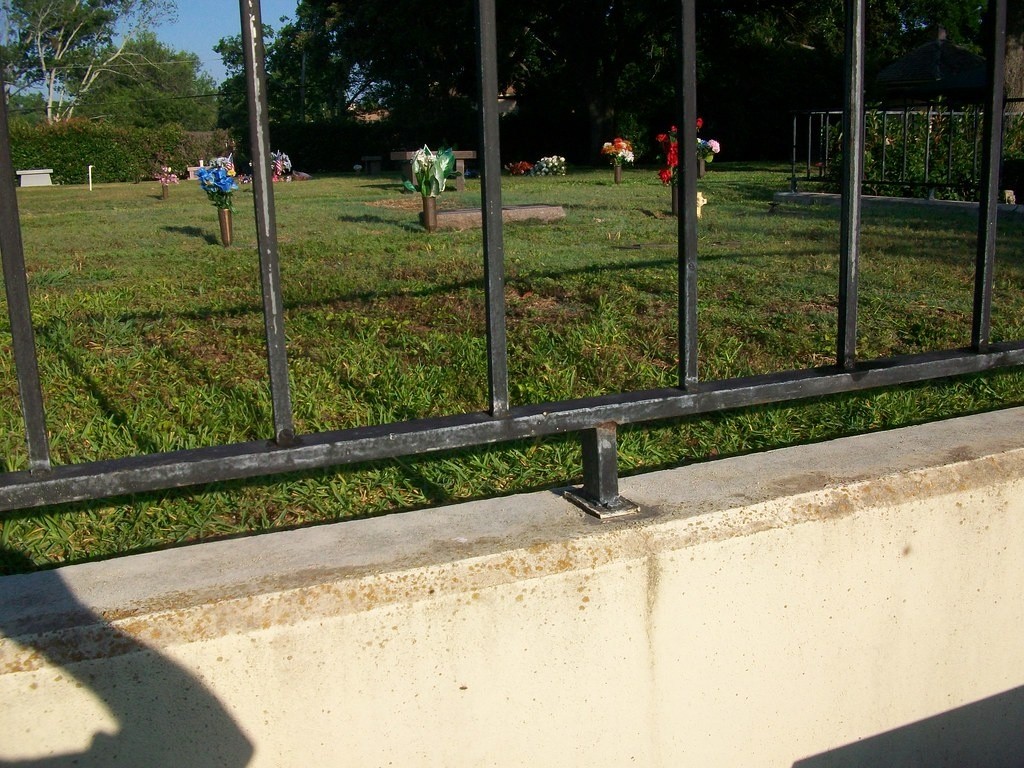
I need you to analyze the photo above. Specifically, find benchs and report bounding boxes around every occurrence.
[391,151,476,192]
[16,169,54,187]
[187,166,210,179]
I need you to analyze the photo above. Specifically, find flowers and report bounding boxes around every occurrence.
[154,166,180,186]
[353,165,363,171]
[653,118,703,187]
[249,150,292,175]
[403,143,464,197]
[601,137,634,166]
[696,138,720,163]
[194,153,240,214]
